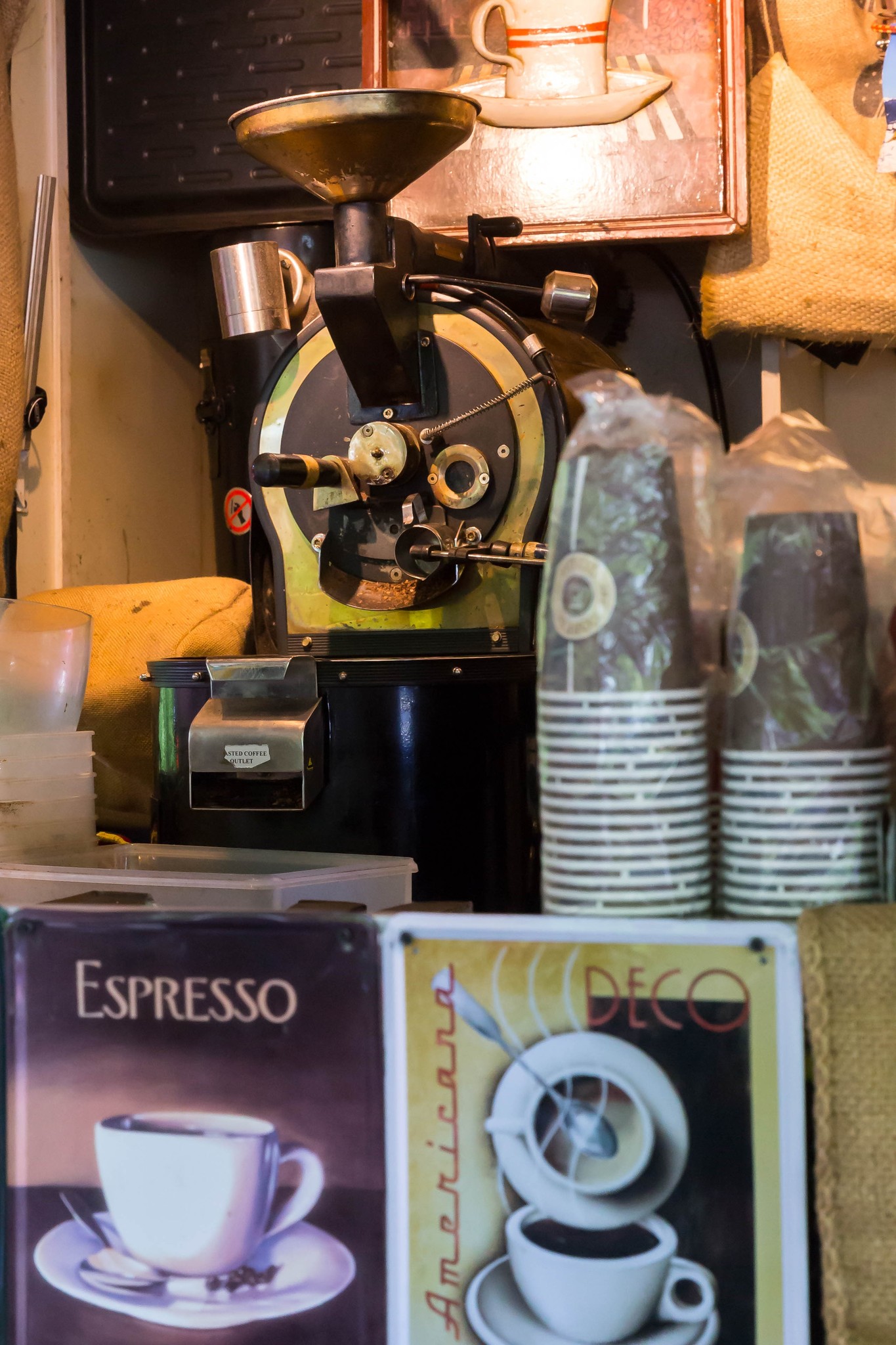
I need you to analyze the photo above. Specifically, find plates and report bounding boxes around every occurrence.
[490,1032,689,1231]
[463,1251,722,1345]
[32,1208,357,1330]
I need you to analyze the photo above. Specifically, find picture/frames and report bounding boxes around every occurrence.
[356,0,752,250]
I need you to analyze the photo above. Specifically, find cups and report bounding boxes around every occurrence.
[483,1067,655,1198]
[93,1109,327,1276]
[1,596,99,737]
[504,1204,717,1345]
[536,447,895,923]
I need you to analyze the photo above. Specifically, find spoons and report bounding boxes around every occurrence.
[432,969,618,1160]
[56,1186,157,1289]
